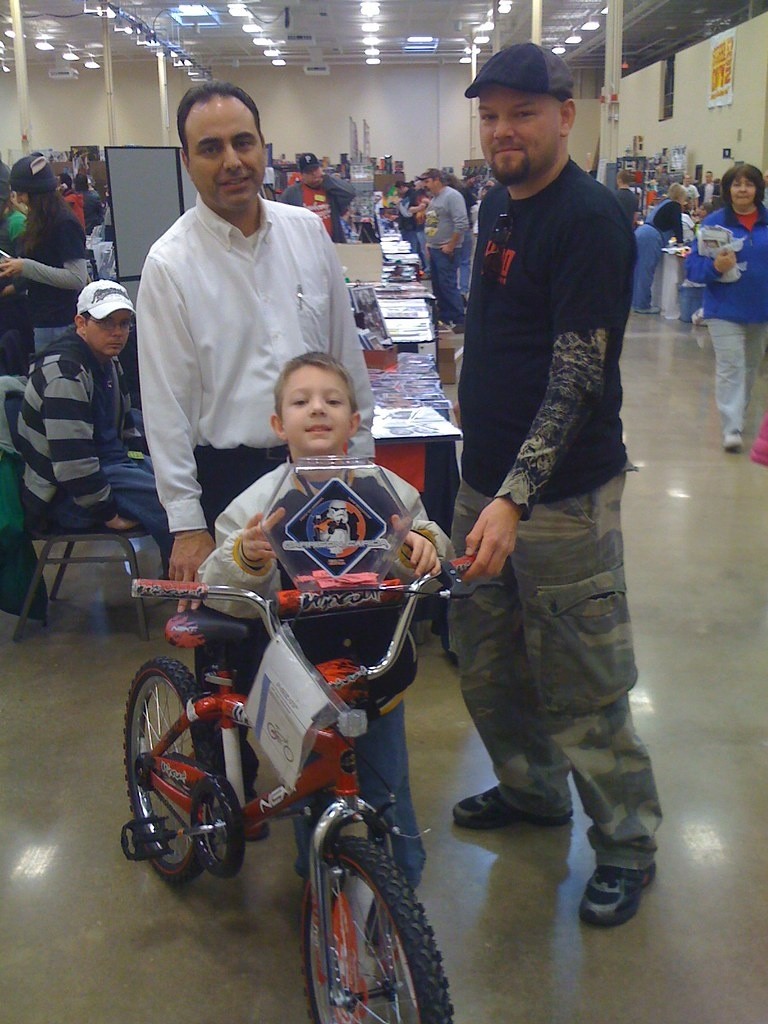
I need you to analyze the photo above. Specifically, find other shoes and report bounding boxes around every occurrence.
[635,305,659,314]
[243,787,269,840]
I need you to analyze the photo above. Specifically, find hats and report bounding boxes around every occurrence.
[60,173,72,188]
[416,168,440,179]
[10,155,57,191]
[299,154,323,172]
[463,43,574,102]
[77,279,136,319]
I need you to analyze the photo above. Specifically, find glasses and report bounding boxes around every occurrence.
[84,318,136,332]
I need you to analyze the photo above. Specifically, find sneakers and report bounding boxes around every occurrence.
[454,788,573,828]
[581,857,658,928]
[723,430,744,453]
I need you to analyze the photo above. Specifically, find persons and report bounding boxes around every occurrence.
[0,147,112,375]
[447,40,666,929]
[277,153,498,333]
[682,162,768,467]
[584,163,768,314]
[15,279,176,581]
[194,352,456,961]
[135,82,375,845]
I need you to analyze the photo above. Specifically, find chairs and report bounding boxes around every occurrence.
[4,377,154,643]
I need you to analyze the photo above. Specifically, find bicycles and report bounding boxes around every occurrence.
[120,556,506,1024]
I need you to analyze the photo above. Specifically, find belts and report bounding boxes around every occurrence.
[201,443,291,461]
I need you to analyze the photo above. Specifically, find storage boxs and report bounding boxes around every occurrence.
[437,329,464,387]
[362,344,398,370]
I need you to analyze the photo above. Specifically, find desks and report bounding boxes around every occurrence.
[367,410,464,646]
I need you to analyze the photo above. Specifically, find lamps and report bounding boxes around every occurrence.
[34,33,55,51]
[551,16,600,54]
[62,43,81,62]
[84,52,101,70]
[84,0,211,82]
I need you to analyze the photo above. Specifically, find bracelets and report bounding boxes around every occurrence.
[173,529,206,540]
